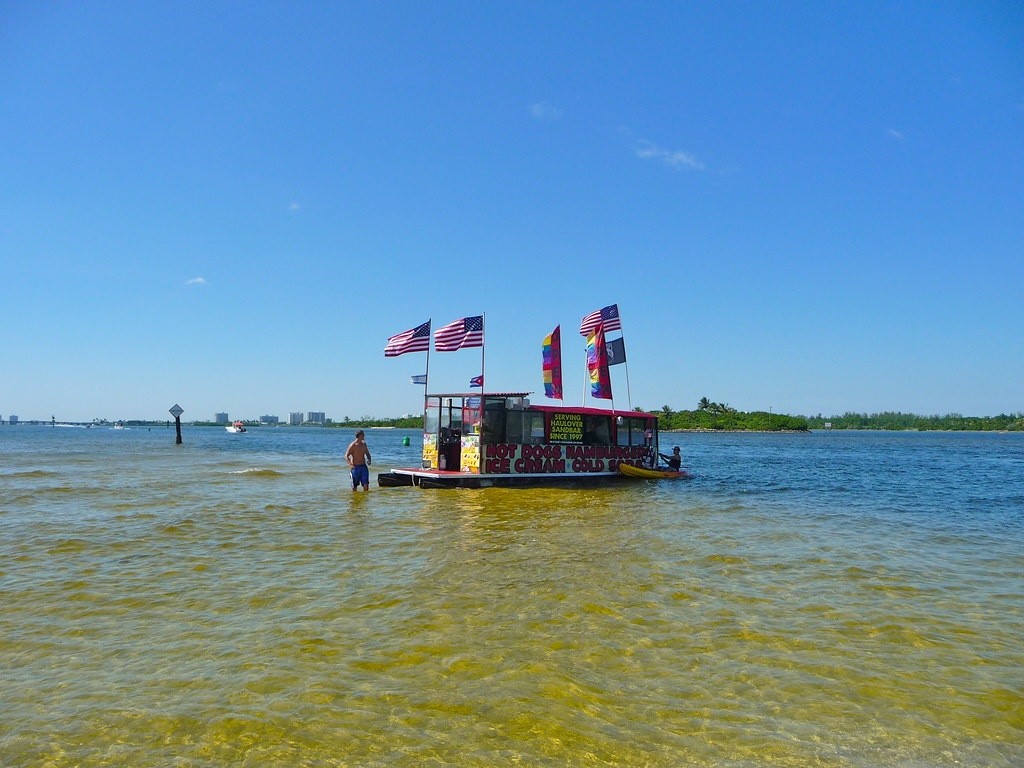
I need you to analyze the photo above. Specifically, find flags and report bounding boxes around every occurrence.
[384,321,429,357]
[410,375,427,385]
[434,315,484,352]
[469,375,483,387]
[541,325,562,399]
[586,321,613,400]
[605,337,626,366]
[579,304,621,336]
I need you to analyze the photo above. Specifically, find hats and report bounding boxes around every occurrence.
[672,446,681,451]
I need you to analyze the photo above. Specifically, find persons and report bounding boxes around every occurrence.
[344,430,372,492]
[656,447,681,473]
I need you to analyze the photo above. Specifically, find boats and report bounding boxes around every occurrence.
[618,462,686,479]
[225,422,247,433]
[114,422,125,429]
[377,304,660,489]
